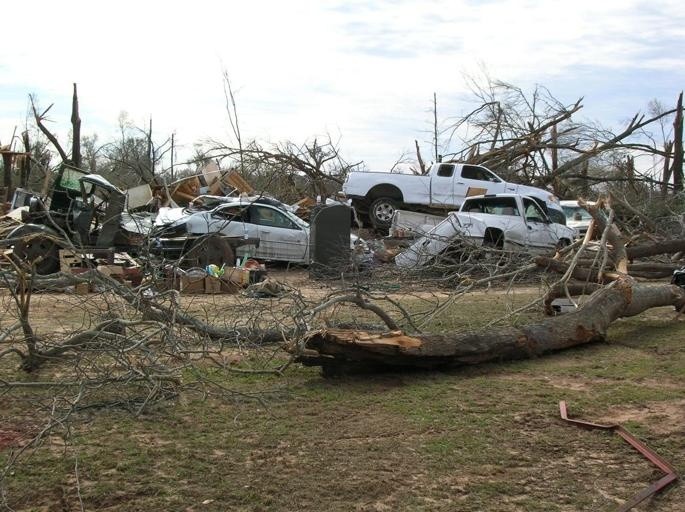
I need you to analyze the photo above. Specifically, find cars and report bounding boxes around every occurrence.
[557,198,603,238]
[153,194,374,271]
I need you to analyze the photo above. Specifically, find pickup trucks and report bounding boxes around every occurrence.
[389,192,574,270]
[338,159,561,226]
